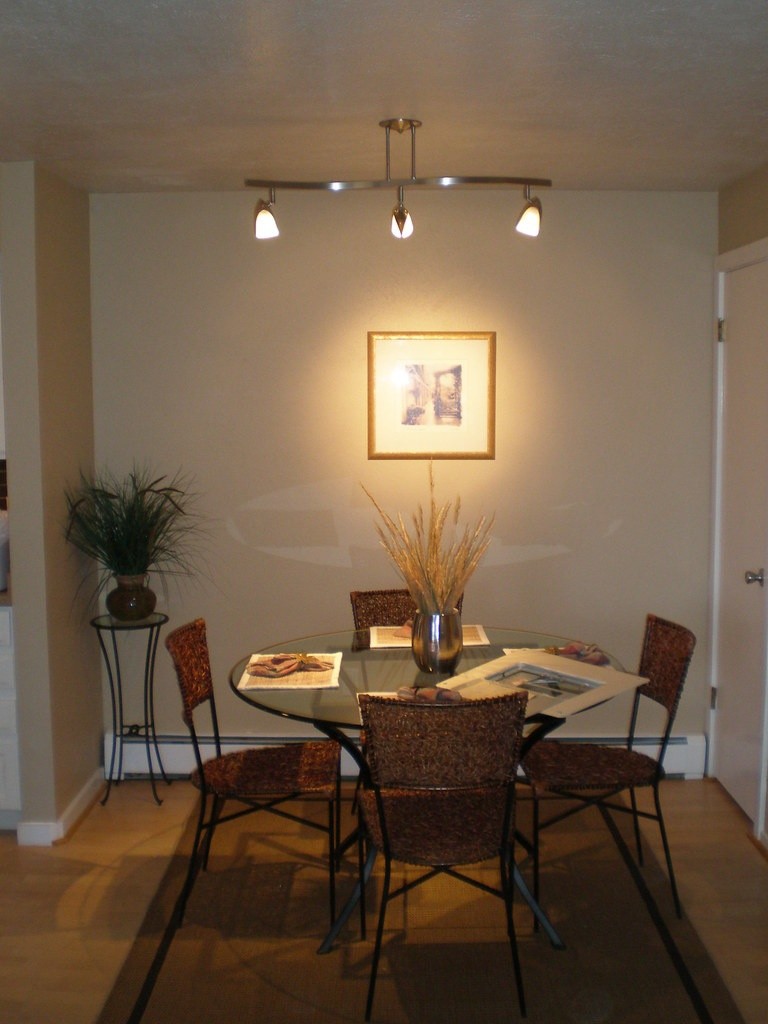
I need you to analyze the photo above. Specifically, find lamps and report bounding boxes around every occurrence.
[241,118,554,240]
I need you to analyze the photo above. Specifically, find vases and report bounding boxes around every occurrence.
[412,609,464,673]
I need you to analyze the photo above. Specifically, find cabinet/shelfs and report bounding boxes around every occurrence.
[89,614,173,806]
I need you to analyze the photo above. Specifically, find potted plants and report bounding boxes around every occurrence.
[64,461,198,621]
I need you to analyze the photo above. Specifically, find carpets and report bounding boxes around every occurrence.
[98,784,745,1024]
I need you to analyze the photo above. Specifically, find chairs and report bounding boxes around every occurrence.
[522,613,697,931]
[348,587,465,819]
[164,615,341,930]
[354,690,530,1022]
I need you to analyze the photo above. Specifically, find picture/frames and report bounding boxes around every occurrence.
[366,331,495,459]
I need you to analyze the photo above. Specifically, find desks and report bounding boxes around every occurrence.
[220,625,628,954]
